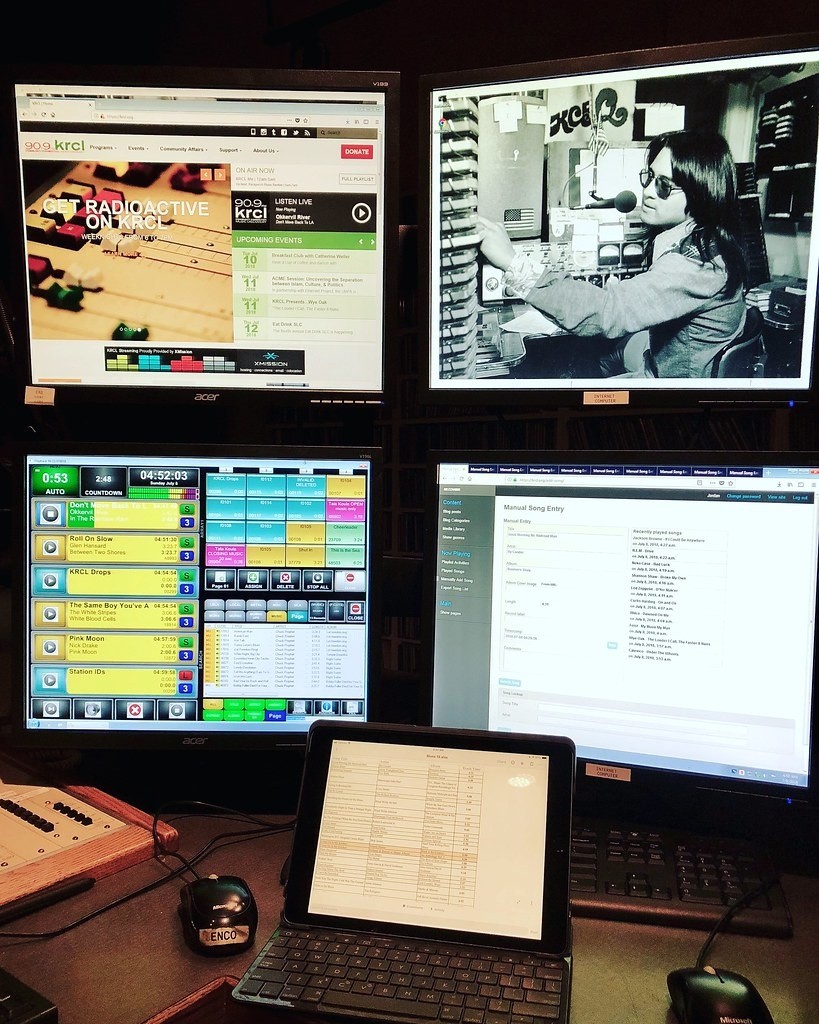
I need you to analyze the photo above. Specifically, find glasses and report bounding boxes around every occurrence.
[639,169,684,200]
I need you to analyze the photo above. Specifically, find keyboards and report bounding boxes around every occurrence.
[569,827,793,941]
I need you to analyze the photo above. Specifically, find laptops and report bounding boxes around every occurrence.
[232,720,575,1024]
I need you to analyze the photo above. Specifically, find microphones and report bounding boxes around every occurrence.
[571,191,637,213]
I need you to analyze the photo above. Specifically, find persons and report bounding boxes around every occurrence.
[475,129,754,380]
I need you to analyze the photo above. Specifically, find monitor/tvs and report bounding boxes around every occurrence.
[1,33,819,843]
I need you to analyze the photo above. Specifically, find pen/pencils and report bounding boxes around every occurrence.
[0,876,95,926]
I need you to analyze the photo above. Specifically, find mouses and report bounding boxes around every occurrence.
[667,966,774,1024]
[179,872,259,958]
